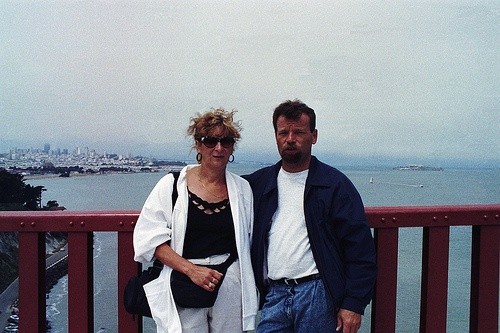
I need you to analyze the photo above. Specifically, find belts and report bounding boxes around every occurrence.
[268,273,321,285]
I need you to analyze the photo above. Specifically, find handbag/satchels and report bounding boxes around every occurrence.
[124,270,160,318]
[170,264,227,306]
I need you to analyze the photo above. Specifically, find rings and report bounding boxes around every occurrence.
[212,278,215,282]
[208,282,211,287]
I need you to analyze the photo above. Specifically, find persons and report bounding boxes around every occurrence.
[133,110,261,333]
[240,100,377,333]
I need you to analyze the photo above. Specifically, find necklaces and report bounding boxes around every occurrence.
[197,166,227,198]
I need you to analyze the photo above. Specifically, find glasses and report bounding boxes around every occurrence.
[196,136,234,149]
